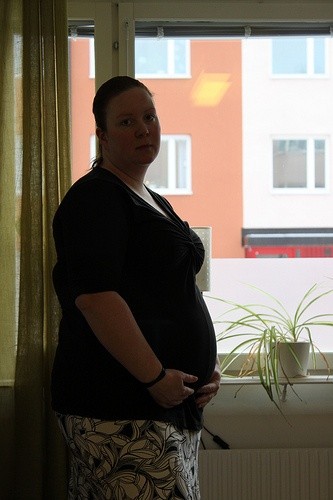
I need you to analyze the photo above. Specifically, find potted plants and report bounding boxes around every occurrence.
[203,280,333,417]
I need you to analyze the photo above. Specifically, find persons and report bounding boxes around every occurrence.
[51,76,221,500]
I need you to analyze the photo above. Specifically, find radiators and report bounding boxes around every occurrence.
[198,447,332,500]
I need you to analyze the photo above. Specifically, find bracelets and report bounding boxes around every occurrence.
[141,368,166,388]
